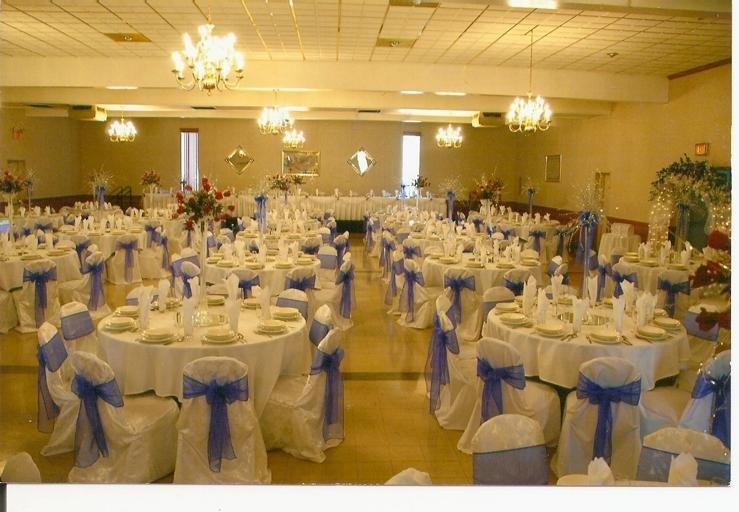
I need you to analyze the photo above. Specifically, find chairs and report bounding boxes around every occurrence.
[1,170,731,486]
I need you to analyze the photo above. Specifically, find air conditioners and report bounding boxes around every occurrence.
[67,105,107,123]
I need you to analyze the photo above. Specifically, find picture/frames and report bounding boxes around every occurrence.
[280,149,321,177]
[544,153,562,184]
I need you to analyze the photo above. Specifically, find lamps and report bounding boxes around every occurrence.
[282,126,305,149]
[435,108,467,150]
[105,103,139,145]
[167,0,246,94]
[255,87,296,136]
[505,30,555,135]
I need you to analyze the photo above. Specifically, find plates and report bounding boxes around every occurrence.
[106,294,301,343]
[623,248,684,271]
[208,227,322,270]
[427,250,539,271]
[494,292,683,345]
[15,225,144,265]
[409,228,486,243]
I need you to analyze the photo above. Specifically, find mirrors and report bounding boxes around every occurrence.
[346,146,376,175]
[223,145,254,175]
